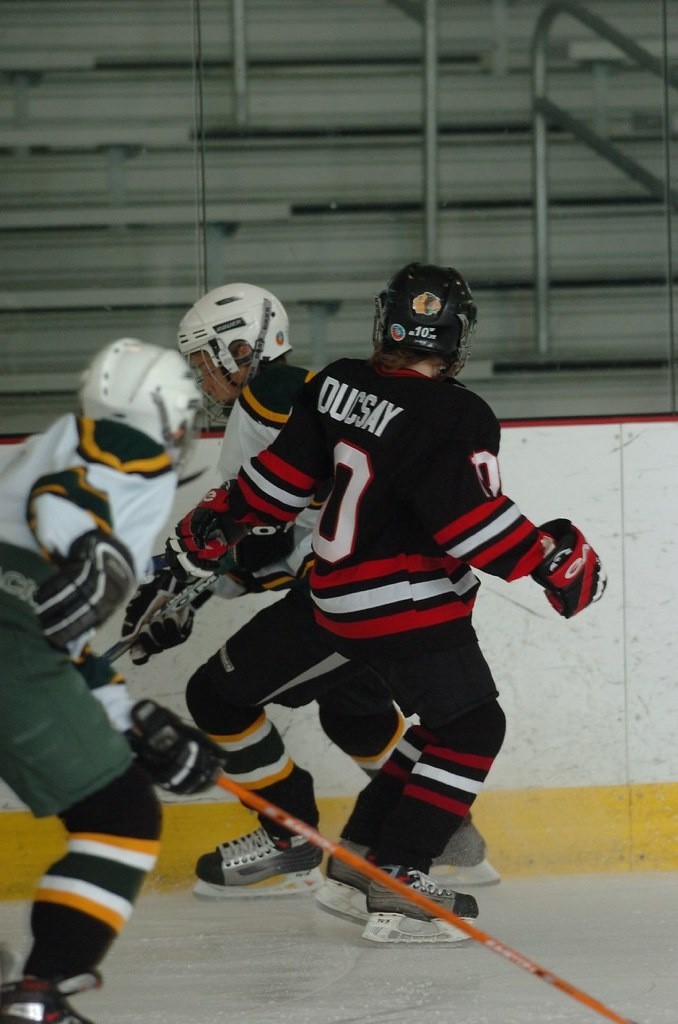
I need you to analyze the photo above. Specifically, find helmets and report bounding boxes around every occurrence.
[80,337,202,474]
[374,263,477,377]
[176,282,291,425]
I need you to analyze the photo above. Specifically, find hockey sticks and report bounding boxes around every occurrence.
[133,727,638,1024]
[98,560,238,665]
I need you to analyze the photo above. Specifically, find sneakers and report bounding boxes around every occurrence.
[313,840,372,925]
[361,866,479,942]
[0,968,104,1024]
[429,822,502,884]
[193,827,324,900]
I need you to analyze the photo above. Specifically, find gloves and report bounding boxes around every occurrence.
[125,700,227,795]
[121,574,212,665]
[164,478,252,583]
[529,518,608,619]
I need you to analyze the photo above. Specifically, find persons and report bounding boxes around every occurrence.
[0,338,227,1024]
[122,262,607,946]
[177,280,500,900]
